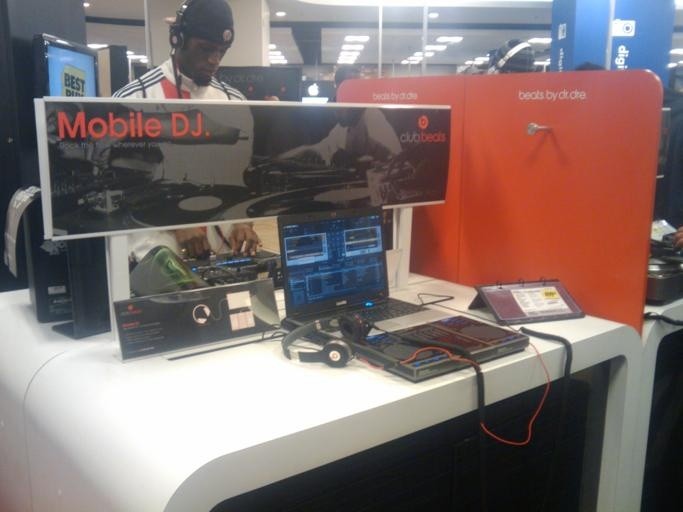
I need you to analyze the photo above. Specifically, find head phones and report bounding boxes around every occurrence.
[282,312,370,367]
[169,0,191,49]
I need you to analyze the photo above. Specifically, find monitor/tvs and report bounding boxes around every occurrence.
[39,33,100,97]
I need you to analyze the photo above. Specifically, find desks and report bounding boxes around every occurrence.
[641,299,683,512]
[22,277,642,512]
[0,271,433,512]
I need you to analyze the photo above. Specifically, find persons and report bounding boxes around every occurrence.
[490,40,534,74]
[279,107,403,170]
[327,67,361,102]
[112,1,264,274]
[673,227,683,250]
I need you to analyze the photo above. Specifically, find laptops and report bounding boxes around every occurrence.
[278,206,458,344]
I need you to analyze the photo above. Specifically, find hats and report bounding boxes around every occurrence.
[164,1,235,45]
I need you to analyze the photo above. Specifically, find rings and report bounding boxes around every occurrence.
[180,248,188,255]
